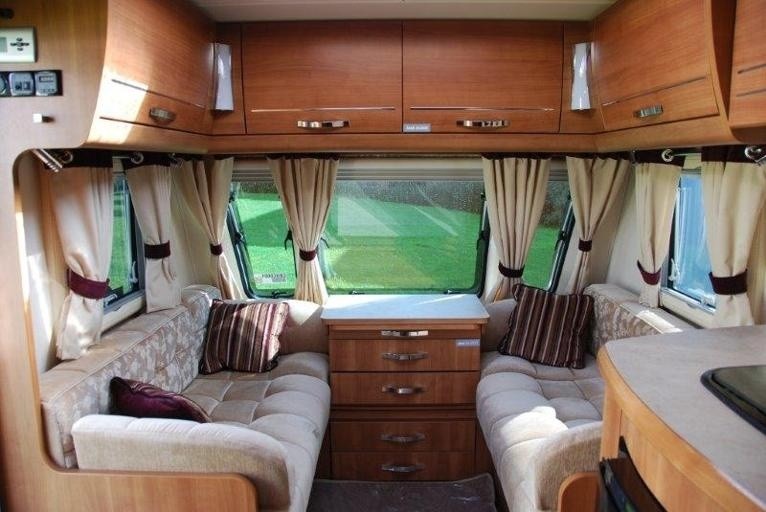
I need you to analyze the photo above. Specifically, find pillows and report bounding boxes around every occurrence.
[198,298,289,374]
[109,375,211,422]
[498,283,595,369]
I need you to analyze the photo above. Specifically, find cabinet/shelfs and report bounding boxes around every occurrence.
[83,0,766,155]
[321,292,491,481]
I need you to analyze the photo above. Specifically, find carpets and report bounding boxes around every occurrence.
[307,473,499,512]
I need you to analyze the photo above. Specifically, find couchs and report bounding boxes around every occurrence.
[36,284,333,512]
[473,283,697,512]
